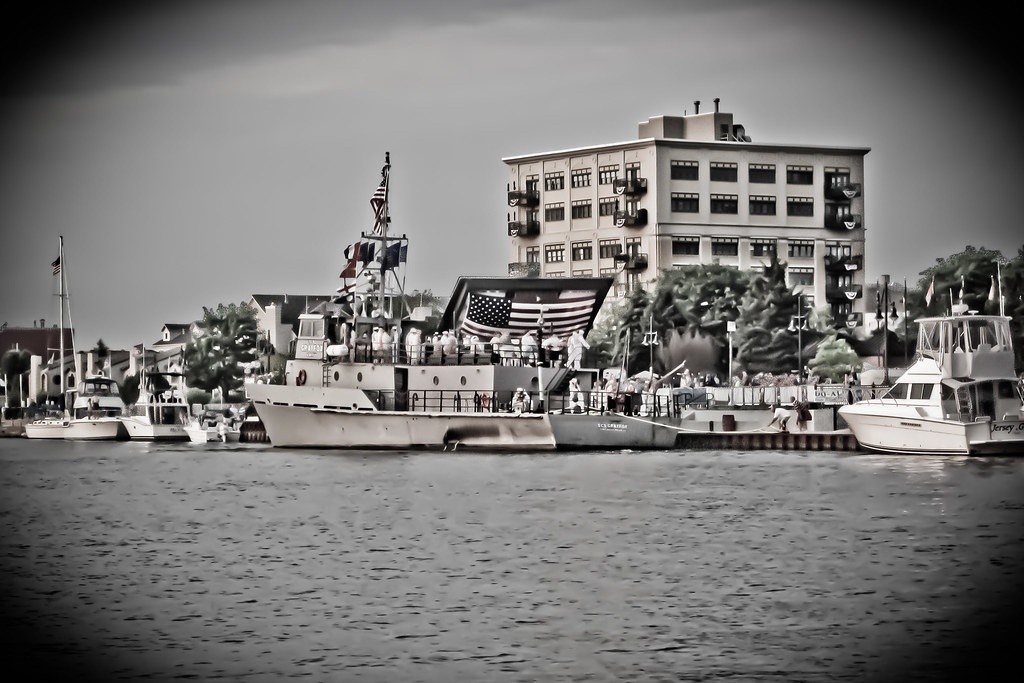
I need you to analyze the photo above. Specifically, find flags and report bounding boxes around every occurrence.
[51,256,60,275]
[462,293,596,343]
[337,234,407,296]
[924,284,935,307]
[371,170,391,235]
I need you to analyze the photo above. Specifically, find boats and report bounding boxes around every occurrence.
[181,385,251,444]
[23,373,123,439]
[837,261,1023,458]
[120,343,194,439]
[243,148,690,452]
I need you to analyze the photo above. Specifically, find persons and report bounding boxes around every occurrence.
[87,398,93,420]
[768,404,790,431]
[355,323,662,417]
[92,392,100,419]
[680,367,855,406]
[180,368,285,443]
[789,396,812,430]
